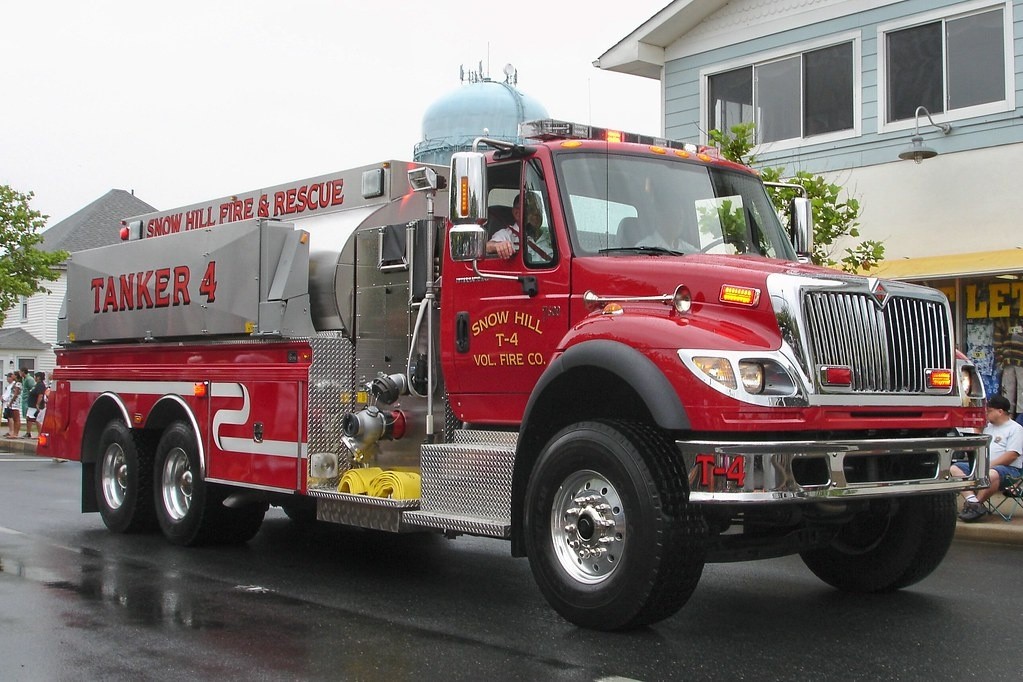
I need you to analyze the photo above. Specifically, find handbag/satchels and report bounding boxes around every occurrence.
[39,394,45,409]
[4,406,9,419]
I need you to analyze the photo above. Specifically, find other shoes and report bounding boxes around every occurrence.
[4,433,10,437]
[21,433,31,438]
[7,435,18,439]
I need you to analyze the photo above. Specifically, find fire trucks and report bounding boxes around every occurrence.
[35,114,994,635]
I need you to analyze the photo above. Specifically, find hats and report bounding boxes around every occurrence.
[987,396,1010,411]
[4,373,12,377]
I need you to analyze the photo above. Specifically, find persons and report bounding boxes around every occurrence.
[635,196,703,255]
[0,367,48,439]
[486,193,553,263]
[950,394,1023,522]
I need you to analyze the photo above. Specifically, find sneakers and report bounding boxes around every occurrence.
[959,501,988,520]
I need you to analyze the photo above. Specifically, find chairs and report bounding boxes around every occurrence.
[974,414,1023,522]
[614,217,658,249]
[486,205,517,241]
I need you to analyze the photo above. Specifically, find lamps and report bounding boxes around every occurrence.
[898,106,952,164]
